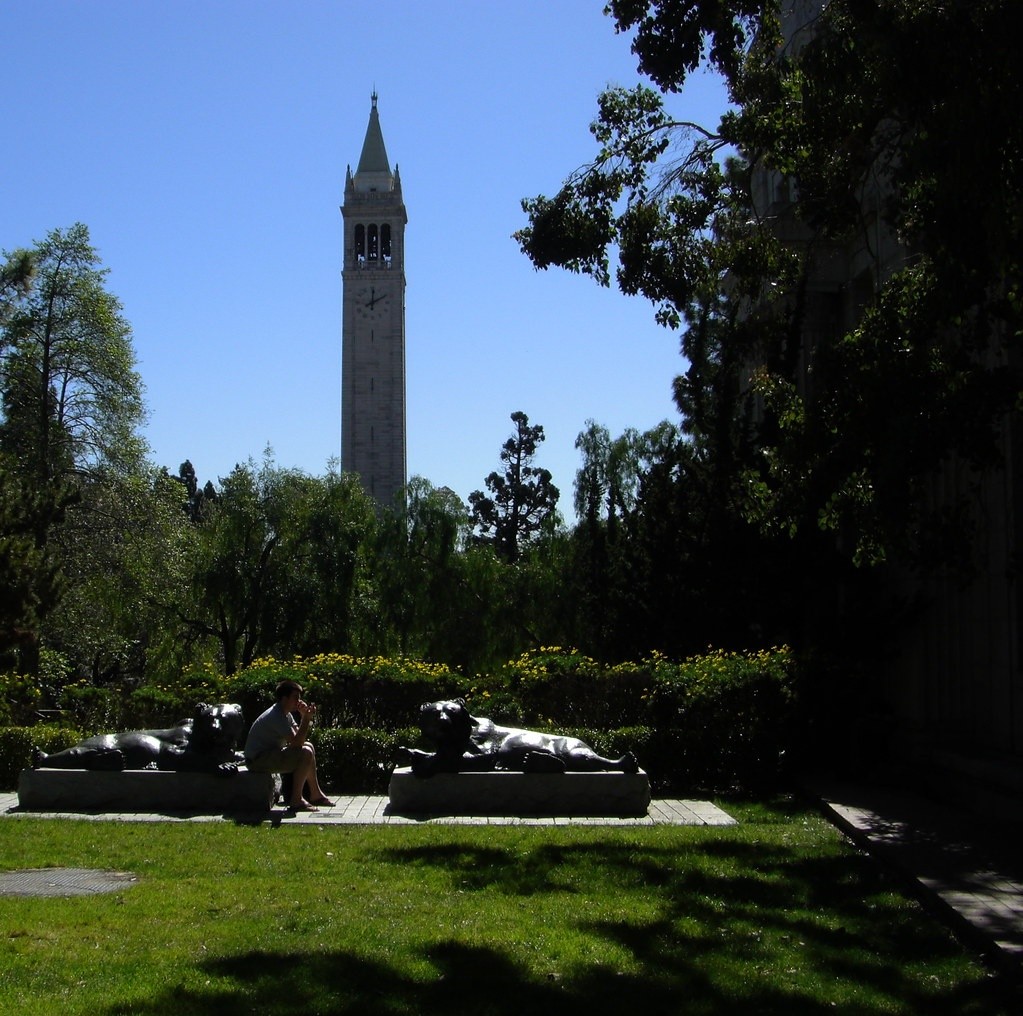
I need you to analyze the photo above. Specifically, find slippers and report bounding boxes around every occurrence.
[287,803,318,813]
[308,796,336,807]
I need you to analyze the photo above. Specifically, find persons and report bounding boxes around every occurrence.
[243,680,338,811]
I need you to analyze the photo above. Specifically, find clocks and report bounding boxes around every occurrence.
[354,286,391,320]
